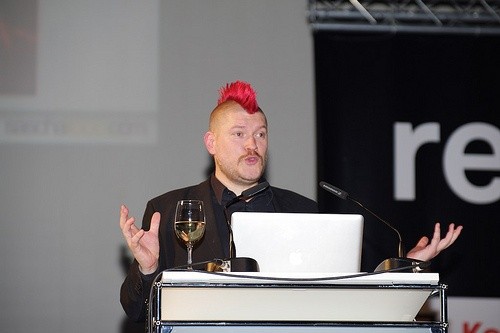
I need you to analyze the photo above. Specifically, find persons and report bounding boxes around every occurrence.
[116,80,463,333]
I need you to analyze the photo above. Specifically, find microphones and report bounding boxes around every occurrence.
[320,182,432,274]
[217,181,269,273]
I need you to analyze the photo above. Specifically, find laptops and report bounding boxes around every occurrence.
[230,211,364,274]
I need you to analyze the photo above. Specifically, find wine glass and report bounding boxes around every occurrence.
[174,200,206,272]
